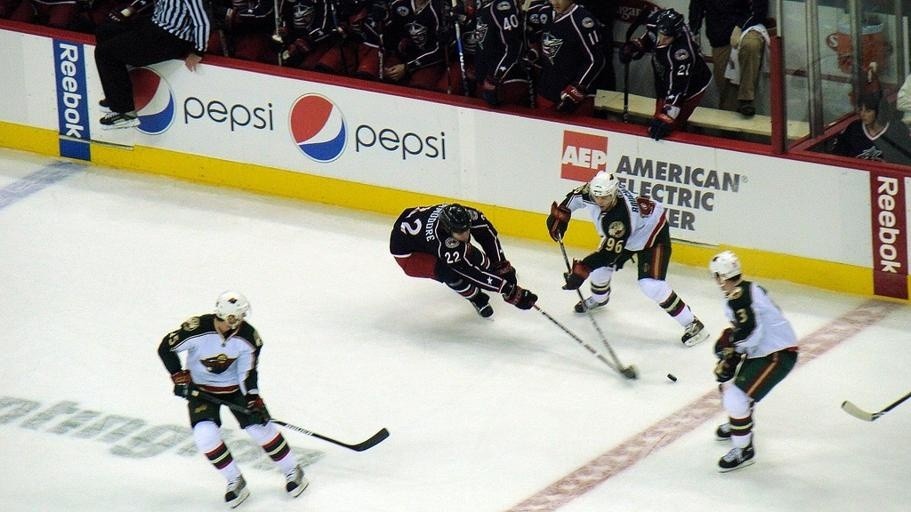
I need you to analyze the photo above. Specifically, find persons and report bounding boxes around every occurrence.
[95,1,211,127]
[155,292,305,502]
[896,73,911,126]
[707,250,798,470]
[3,0,613,116]
[688,0,770,116]
[620,6,714,141]
[390,201,539,317]
[834,89,910,165]
[546,170,705,344]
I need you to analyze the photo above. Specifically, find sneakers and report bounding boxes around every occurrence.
[737,95,757,120]
[682,314,705,345]
[715,413,756,469]
[468,295,496,317]
[99,106,141,131]
[284,463,304,492]
[223,473,247,502]
[576,290,610,311]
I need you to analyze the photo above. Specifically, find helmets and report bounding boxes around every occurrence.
[588,170,621,202]
[213,290,251,329]
[649,6,686,49]
[439,202,473,238]
[710,247,744,282]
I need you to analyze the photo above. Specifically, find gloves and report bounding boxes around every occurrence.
[556,77,591,118]
[712,328,742,383]
[495,261,518,285]
[546,201,571,239]
[563,254,590,289]
[502,284,537,309]
[619,37,645,65]
[650,101,683,143]
[245,393,270,425]
[173,370,201,399]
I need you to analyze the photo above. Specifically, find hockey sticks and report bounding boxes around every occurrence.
[557,232,636,378]
[188,389,389,451]
[841,393,911,421]
